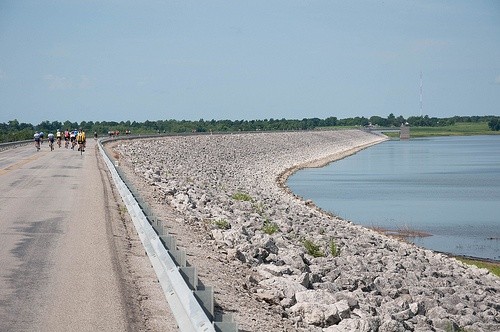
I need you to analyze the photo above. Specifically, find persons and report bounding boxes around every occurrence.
[108,130,119,136]
[64,129,86,152]
[126,129,131,136]
[48,130,54,150]
[39,131,44,143]
[56,129,62,147]
[34,131,40,149]
[94,131,97,138]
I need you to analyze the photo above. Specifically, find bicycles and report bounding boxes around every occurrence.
[39,138,44,144]
[71,139,76,150]
[64,136,69,149]
[57,138,61,148]
[77,140,86,155]
[48,139,54,151]
[34,139,40,151]
